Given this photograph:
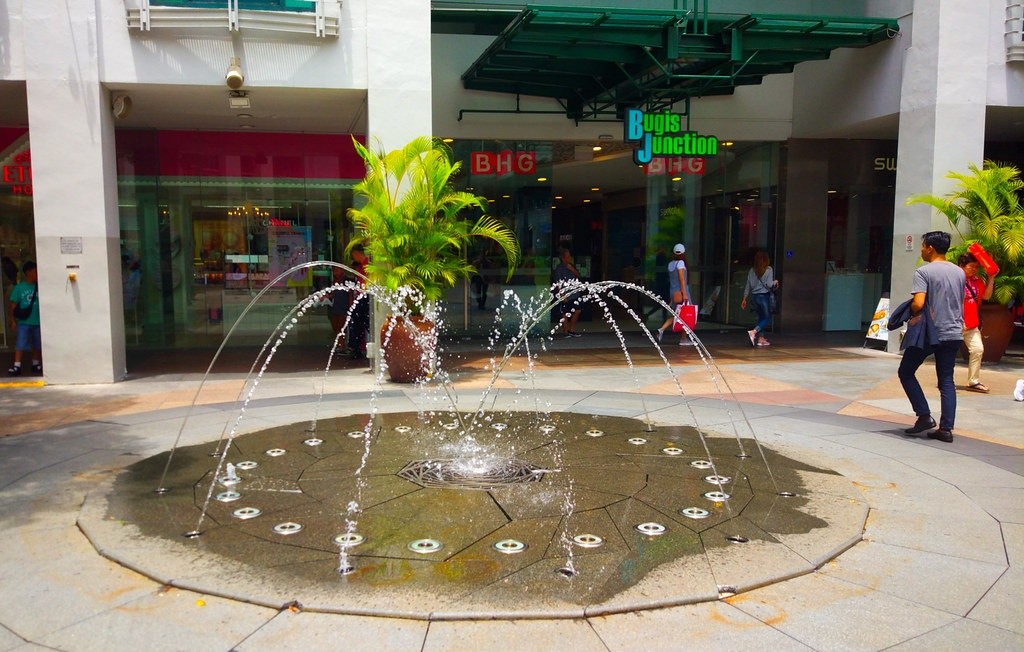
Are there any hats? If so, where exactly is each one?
[672,244,686,255]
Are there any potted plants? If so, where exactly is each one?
[904,154,1024,361]
[343,134,521,379]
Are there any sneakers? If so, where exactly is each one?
[655,327,664,342]
[746,330,756,346]
[757,340,771,347]
[678,338,694,346]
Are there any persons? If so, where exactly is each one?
[553,248,582,339]
[6,260,42,375]
[1014,379,1024,401]
[657,245,699,346]
[952,251,1000,394]
[883,231,965,443]
[218,251,227,267]
[120,242,142,326]
[740,250,779,347]
[1,256,18,348]
[325,263,353,353]
[228,251,243,289]
[642,251,670,323]
[314,265,323,292]
[352,261,361,278]
[345,243,369,360]
[199,246,222,324]
[471,250,491,310]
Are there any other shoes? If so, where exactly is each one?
[30,363,43,374]
[8,364,21,376]
[338,346,355,358]
[563,332,572,338]
[569,330,581,337]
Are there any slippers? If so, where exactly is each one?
[967,382,988,395]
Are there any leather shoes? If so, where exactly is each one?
[905,415,936,434]
[926,427,954,444]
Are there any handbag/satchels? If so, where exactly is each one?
[673,290,689,304]
[768,289,780,317]
[673,299,698,334]
[13,301,33,321]
